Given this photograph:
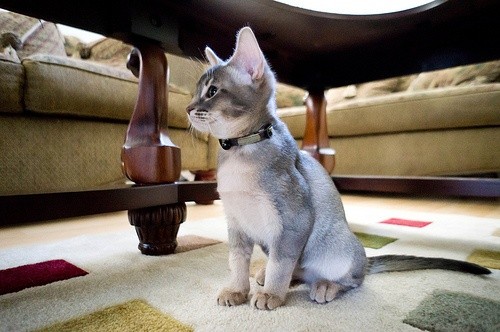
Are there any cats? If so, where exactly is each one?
[185,26,493,312]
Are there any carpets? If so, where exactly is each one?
[0,203,500,332]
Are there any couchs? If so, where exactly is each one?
[0,9,500,205]
